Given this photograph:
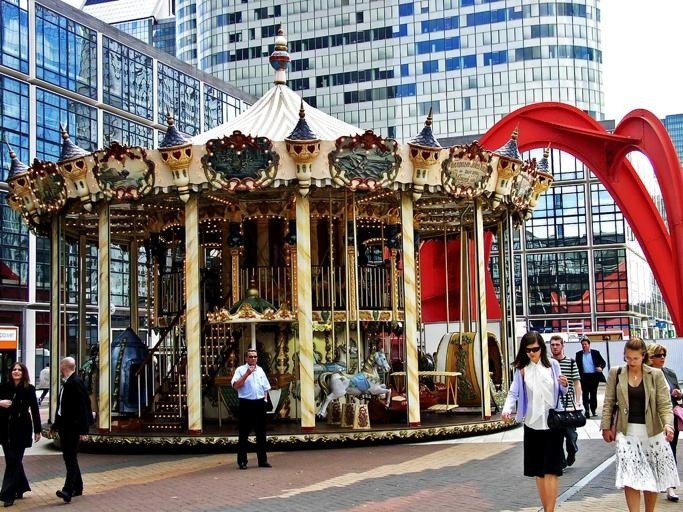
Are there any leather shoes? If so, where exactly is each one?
[4,493,23,507]
[667,490,679,502]
[585,412,590,419]
[239,463,247,470]
[591,409,598,416]
[56,491,71,502]
[259,464,272,467]
[72,490,82,497]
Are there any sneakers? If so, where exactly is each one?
[567,452,575,466]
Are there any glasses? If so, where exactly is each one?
[625,352,646,362]
[525,347,540,353]
[652,354,666,358]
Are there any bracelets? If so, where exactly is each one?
[264,399,268,403]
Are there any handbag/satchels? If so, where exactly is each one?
[610,404,619,441]
[673,404,683,431]
[547,409,586,429]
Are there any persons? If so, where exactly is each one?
[600,337,675,512]
[231,348,272,470]
[549,336,582,466]
[501,331,569,512]
[646,342,681,502]
[38,363,50,405]
[49,356,93,504]
[575,338,606,419]
[0,362,43,508]
